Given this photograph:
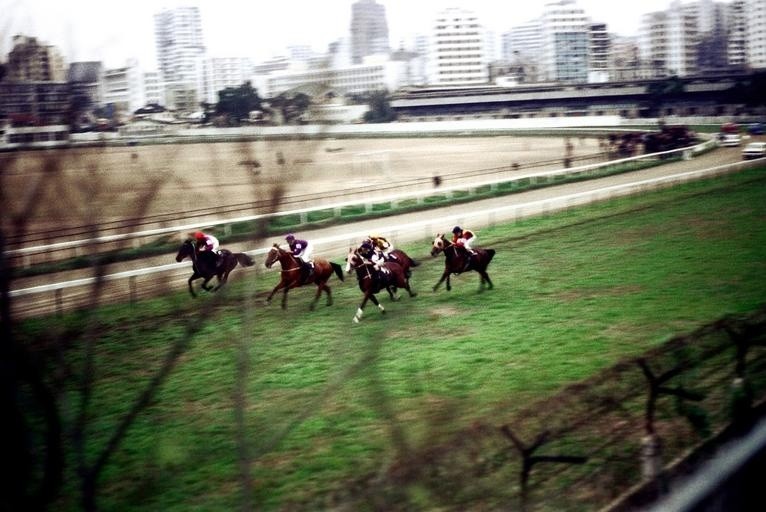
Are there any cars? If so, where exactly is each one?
[717,134,742,147]
[746,122,764,135]
[720,122,742,133]
[741,142,766,158]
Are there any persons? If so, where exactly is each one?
[285,235,315,269]
[362,240,385,271]
[193,231,224,269]
[368,236,393,261]
[446,226,476,254]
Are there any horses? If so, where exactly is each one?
[176,239,255,299]
[262,241,344,311]
[430,233,496,294]
[345,239,421,324]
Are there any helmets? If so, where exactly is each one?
[285,235,295,240]
[194,232,204,238]
[360,232,378,250]
[452,226,461,233]
